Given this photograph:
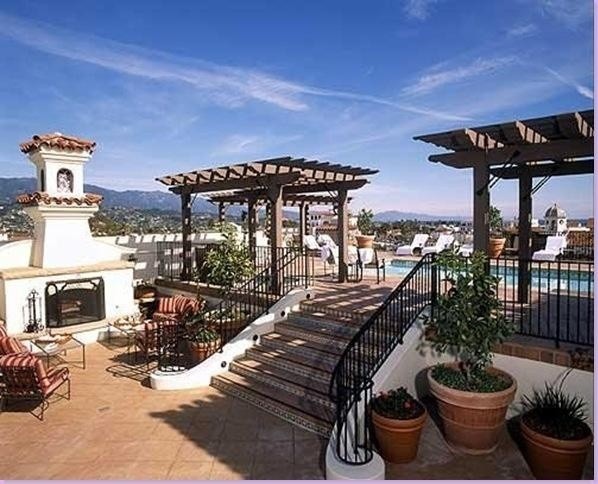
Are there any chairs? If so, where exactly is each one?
[0,324,71,421]
[505,236,532,258]
[532,236,567,261]
[304,234,385,284]
[395,234,474,257]
[133,296,206,371]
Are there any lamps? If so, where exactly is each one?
[27,288,44,333]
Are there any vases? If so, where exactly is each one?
[369,402,427,463]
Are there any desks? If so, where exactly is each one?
[30,333,86,369]
[108,316,146,362]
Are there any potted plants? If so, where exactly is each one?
[199,218,256,346]
[186,330,222,363]
[354,209,377,248]
[417,239,520,457]
[507,367,593,480]
[487,205,507,258]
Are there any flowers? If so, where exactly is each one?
[371,386,418,417]
